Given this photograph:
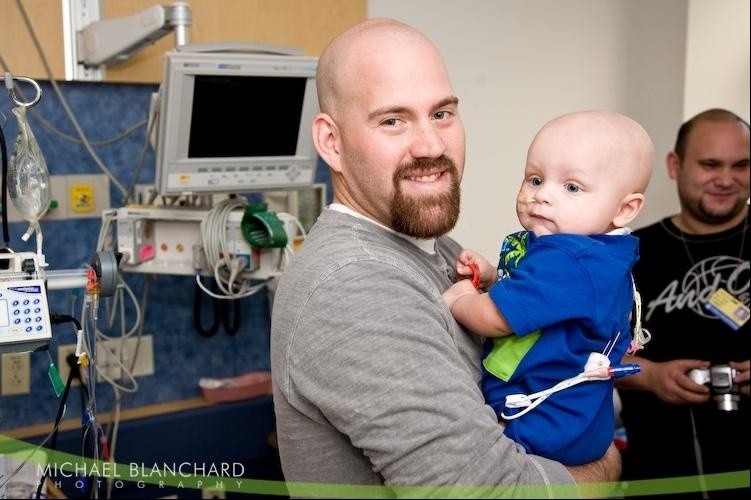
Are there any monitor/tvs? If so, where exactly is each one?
[154,50,322,196]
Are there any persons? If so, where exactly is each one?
[440,110,656,467]
[271,18,623,500]
[619,108,750,500]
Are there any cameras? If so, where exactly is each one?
[686,363,741,417]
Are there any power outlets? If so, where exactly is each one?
[97,340,122,383]
[58,343,89,387]
[1,351,30,396]
